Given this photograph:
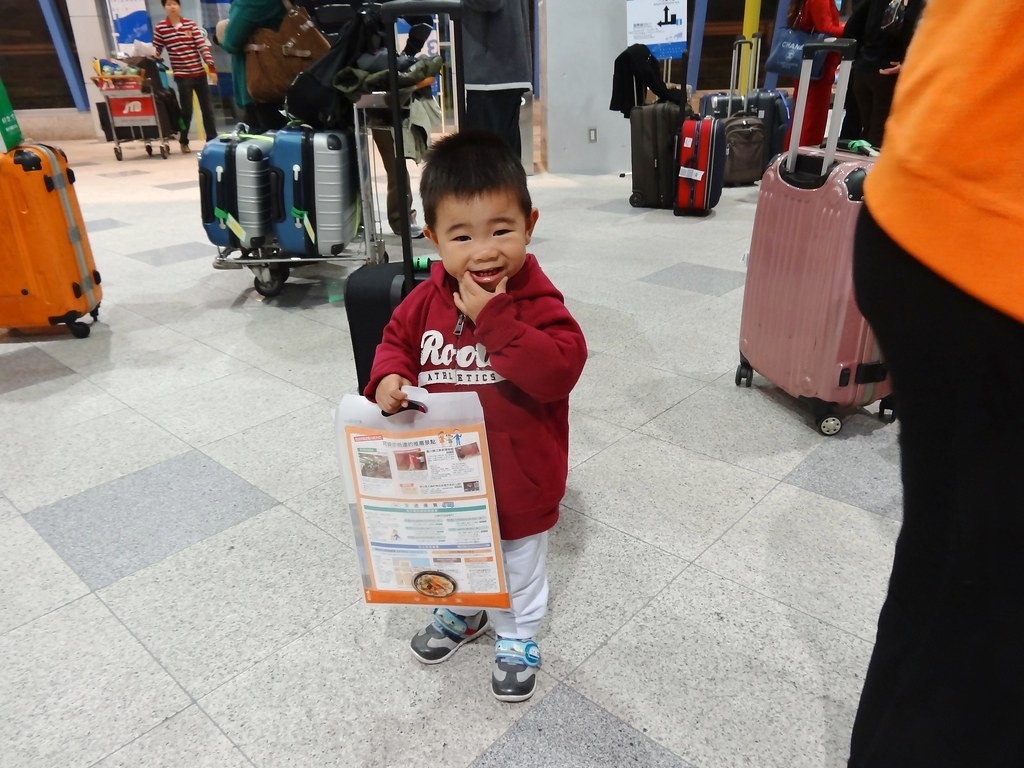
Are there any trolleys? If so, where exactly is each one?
[90,75,180,161]
[210,74,437,296]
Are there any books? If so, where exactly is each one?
[346,425,509,613]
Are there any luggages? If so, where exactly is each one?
[0,140,101,338]
[201,120,360,258]
[737,42,901,438]
[629,36,791,217]
[119,91,181,138]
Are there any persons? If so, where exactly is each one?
[213,0,426,272]
[457,1,534,162]
[360,124,587,705]
[144,0,217,154]
[782,0,848,153]
[849,0,1024,768]
[841,0,926,141]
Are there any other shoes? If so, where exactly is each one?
[397,225,427,238]
[181,141,190,153]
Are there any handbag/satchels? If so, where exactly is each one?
[242,0,334,105]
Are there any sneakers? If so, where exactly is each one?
[410,606,490,665]
[491,635,540,703]
